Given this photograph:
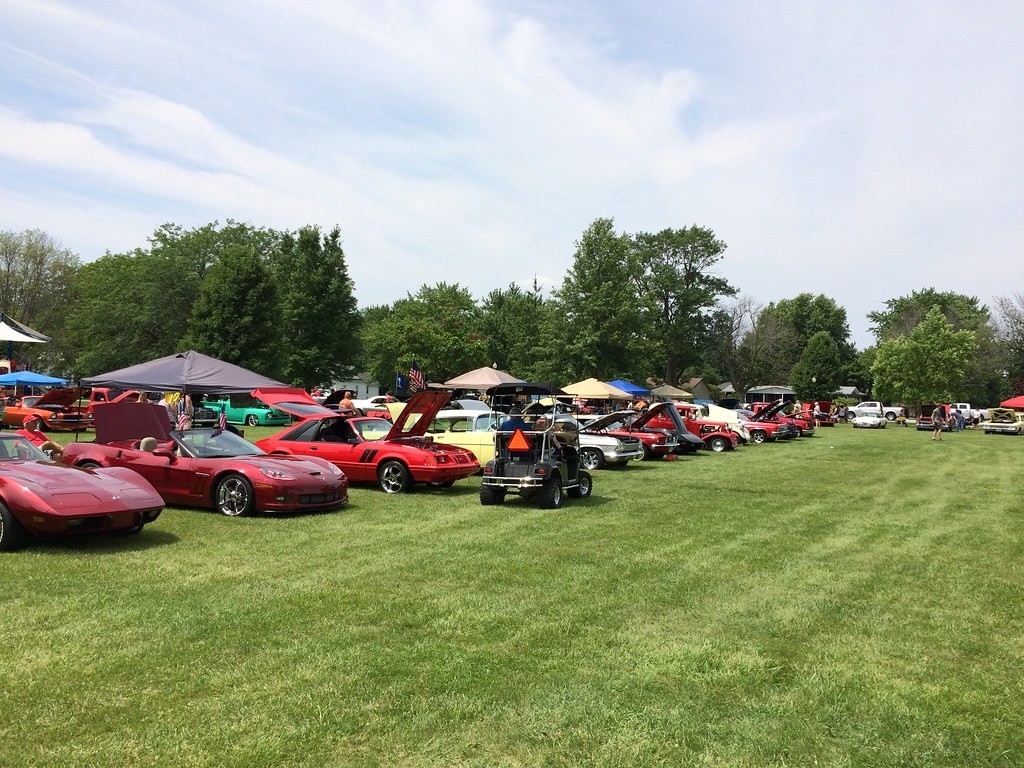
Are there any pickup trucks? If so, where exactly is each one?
[949,403,991,423]
[845,402,903,421]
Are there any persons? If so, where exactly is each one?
[338,391,362,417]
[972,408,980,431]
[13,415,64,463]
[386,391,397,403]
[613,400,634,412]
[500,407,532,431]
[536,419,564,460]
[310,387,322,397]
[134,390,194,430]
[778,398,848,428]
[930,403,946,440]
[948,412,965,433]
[897,405,908,428]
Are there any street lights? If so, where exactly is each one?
[812,377,816,401]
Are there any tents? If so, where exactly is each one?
[443,366,528,409]
[76,349,292,443]
[549,377,633,441]
[650,385,694,418]
[605,380,651,412]
[0,371,69,396]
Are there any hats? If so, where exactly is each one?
[23,415,39,424]
[538,416,546,423]
[814,402,819,405]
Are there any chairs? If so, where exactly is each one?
[140,436,156,452]
[321,427,336,442]
[176,438,194,458]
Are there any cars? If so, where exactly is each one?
[540,410,643,470]
[916,404,952,431]
[648,403,752,452]
[316,390,400,416]
[851,411,887,428]
[88,387,219,428]
[362,398,562,468]
[805,402,835,427]
[982,408,1024,436]
[745,402,815,438]
[2,388,94,432]
[584,402,705,461]
[200,394,291,427]
[730,399,791,445]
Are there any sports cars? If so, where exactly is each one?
[61,403,348,517]
[0,432,166,552]
[250,387,481,494]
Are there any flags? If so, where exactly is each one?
[397,374,407,390]
[408,359,428,392]
